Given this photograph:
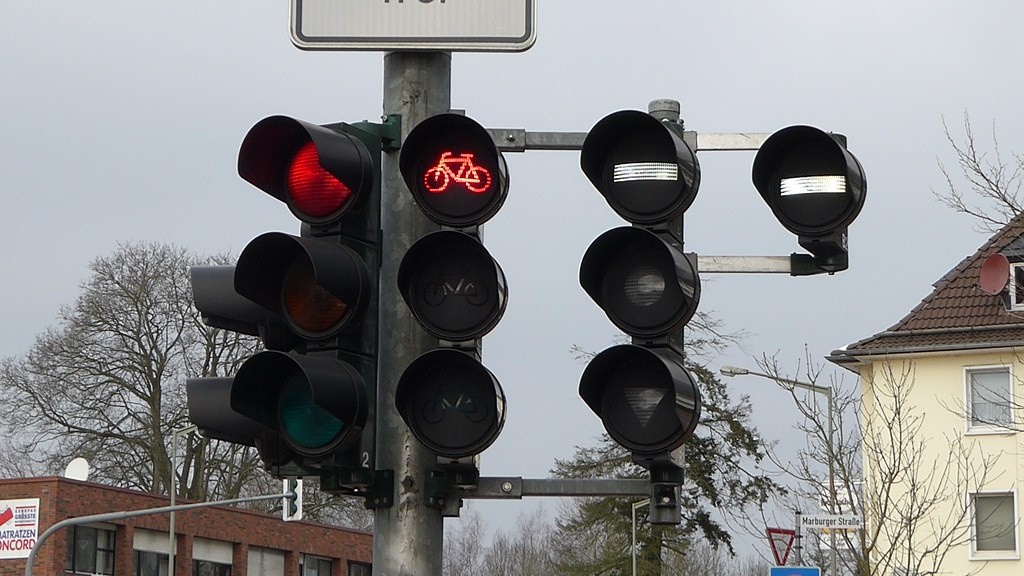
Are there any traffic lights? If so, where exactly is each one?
[185,265,294,469]
[399,112,509,457]
[228,115,376,464]
[751,125,869,239]
[581,109,702,455]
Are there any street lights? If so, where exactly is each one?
[720,364,837,575]
